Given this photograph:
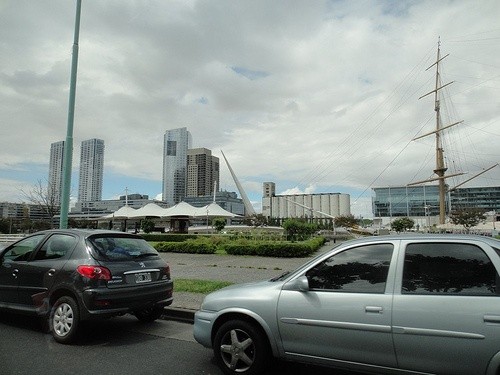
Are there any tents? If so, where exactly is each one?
[102,202,244,233]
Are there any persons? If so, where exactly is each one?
[334,231,337,243]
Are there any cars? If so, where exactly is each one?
[193,234,500,375]
[0,228,174,344]
